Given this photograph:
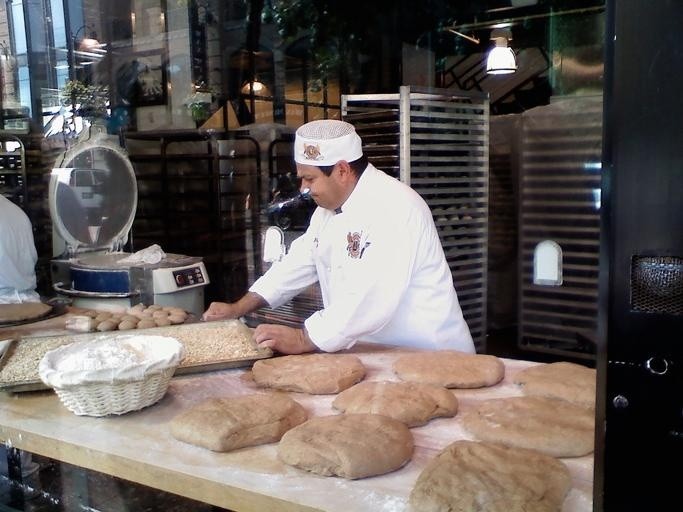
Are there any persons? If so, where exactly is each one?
[196,119,477,359]
[0,194,42,306]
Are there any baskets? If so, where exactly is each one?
[37,334,182,418]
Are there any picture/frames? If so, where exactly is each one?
[109,47,169,109]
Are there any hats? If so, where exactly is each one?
[293,118,363,168]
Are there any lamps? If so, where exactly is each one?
[486,25,519,76]
